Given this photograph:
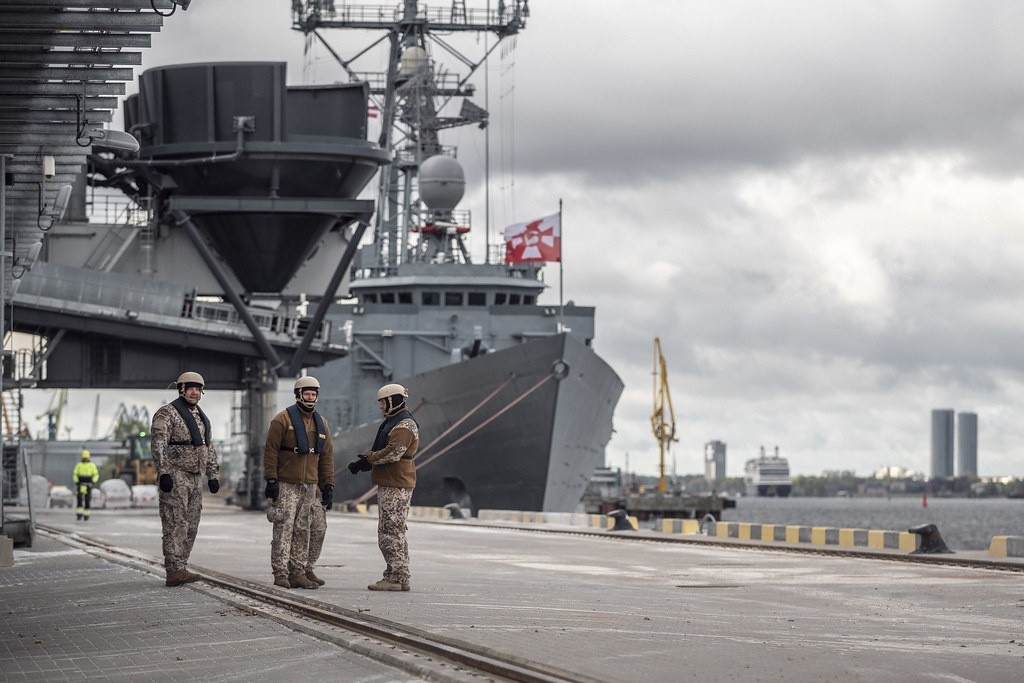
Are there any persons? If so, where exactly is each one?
[73,450,99,520]
[150,372,220,587]
[264,377,336,589]
[348,383,419,592]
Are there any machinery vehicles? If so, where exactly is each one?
[113,434,158,486]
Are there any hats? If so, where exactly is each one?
[81,450,90,459]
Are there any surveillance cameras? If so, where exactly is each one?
[42,155,56,179]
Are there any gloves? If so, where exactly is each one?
[208,480,219,493]
[348,455,372,474]
[159,474,173,493]
[320,484,334,510]
[265,478,279,502]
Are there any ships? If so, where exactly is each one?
[745,446,792,497]
[291,0,627,510]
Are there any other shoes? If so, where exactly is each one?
[367,578,402,591]
[274,575,291,588]
[77,515,82,520]
[305,571,325,586]
[83,516,88,520]
[401,580,410,591]
[166,566,201,586]
[288,573,319,589]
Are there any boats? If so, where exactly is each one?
[578,338,735,522]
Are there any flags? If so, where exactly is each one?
[504,212,561,263]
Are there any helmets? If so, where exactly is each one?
[294,376,320,390]
[177,372,204,387]
[377,384,408,400]
